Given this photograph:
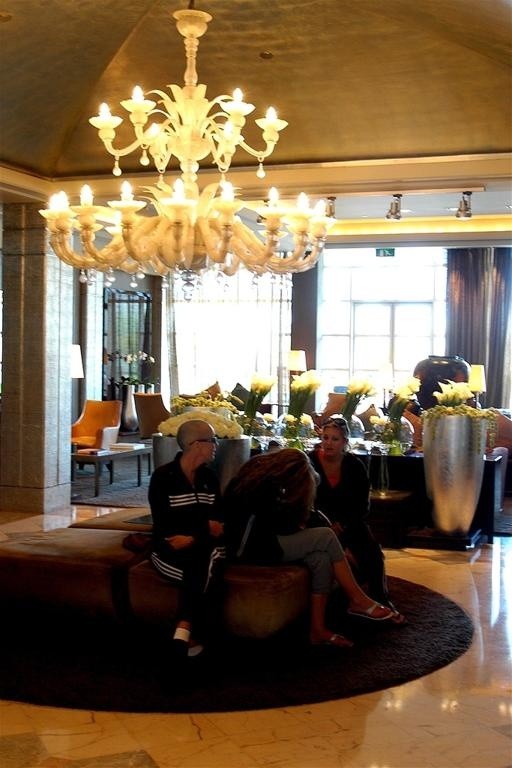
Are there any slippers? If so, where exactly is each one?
[311,634,354,646]
[347,602,408,624]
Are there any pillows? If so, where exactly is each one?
[204,381,221,401]
[226,382,251,403]
[401,410,421,446]
[467,365,486,410]
[322,393,348,415]
[358,404,380,430]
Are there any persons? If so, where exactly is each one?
[306,419,406,626]
[148,419,228,670]
[220,447,394,648]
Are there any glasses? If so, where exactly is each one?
[189,437,219,446]
[321,417,349,432]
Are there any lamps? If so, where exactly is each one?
[255,200,269,224]
[285,350,307,376]
[385,194,404,219]
[38,1,337,302]
[454,191,474,217]
[72,345,85,379]
[325,197,336,220]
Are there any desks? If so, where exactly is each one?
[73,441,154,497]
[250,435,503,545]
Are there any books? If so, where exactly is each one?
[77,448,110,454]
[109,442,146,450]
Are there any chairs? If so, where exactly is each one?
[133,393,174,459]
[485,406,512,459]
[73,400,124,484]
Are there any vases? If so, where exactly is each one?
[123,383,155,431]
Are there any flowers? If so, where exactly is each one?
[111,347,160,389]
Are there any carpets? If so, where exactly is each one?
[1,572,475,714]
